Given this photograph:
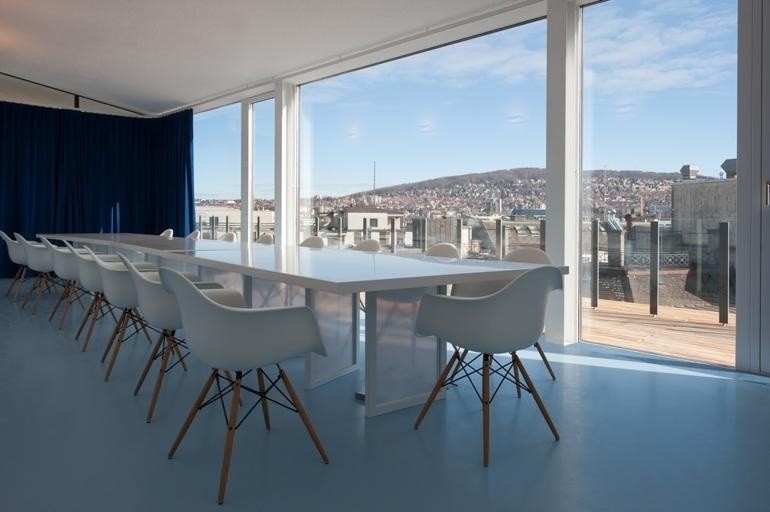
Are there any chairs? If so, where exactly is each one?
[40,235,117,327]
[1,232,62,301]
[82,244,190,382]
[14,232,85,315]
[413,266,564,468]
[158,228,461,310]
[158,267,330,504]
[114,250,245,422]
[62,240,152,352]
[451,245,555,401]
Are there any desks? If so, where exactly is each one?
[37,232,564,419]
